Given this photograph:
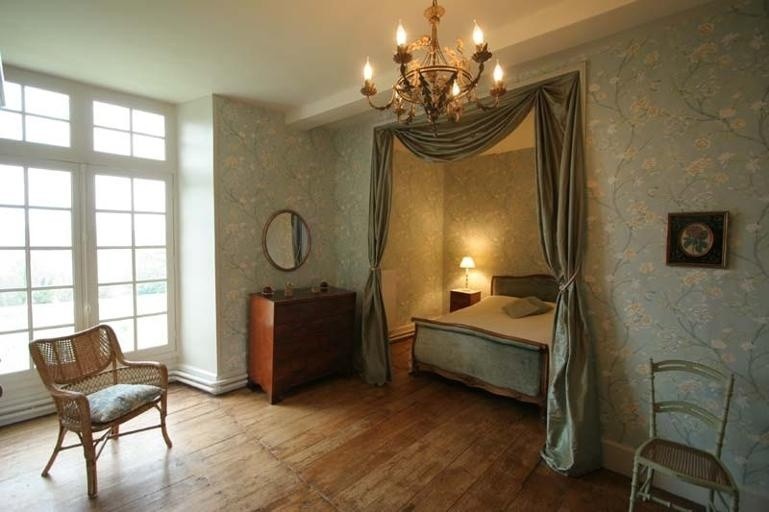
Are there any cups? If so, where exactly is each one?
[262,281,331,296]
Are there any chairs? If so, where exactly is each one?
[27,322,173,500]
[627,356,740,512]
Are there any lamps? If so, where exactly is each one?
[358,2,508,140]
[458,255,476,292]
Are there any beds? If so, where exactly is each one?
[408,274,560,426]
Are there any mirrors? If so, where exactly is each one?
[261,208,313,273]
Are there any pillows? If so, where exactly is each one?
[501,294,555,320]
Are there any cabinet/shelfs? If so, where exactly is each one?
[246,285,358,406]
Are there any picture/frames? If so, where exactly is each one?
[664,209,731,270]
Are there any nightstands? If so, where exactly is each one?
[449,288,482,313]
